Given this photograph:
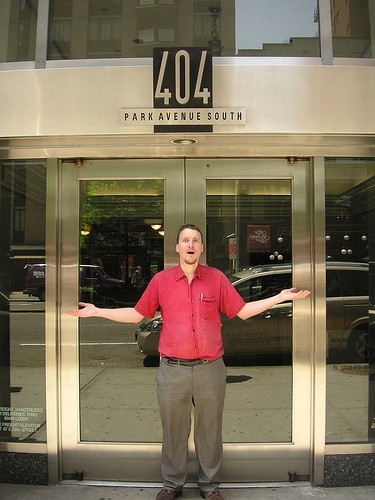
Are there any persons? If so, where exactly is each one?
[66,224,313,500]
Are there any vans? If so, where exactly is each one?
[133,258,371,360]
[22,263,124,302]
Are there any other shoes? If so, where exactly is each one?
[199,489,224,500]
[155,487,183,500]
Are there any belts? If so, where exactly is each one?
[163,355,207,367]
